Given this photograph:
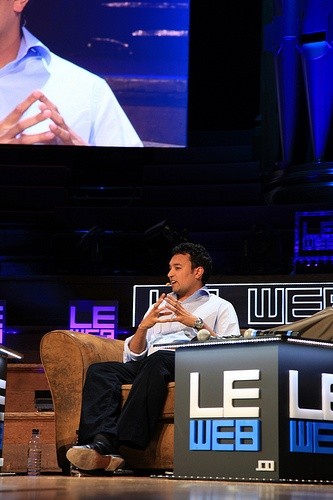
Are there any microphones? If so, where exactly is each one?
[244,329,300,338]
[197,329,226,341]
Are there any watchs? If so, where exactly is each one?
[193,317,204,332]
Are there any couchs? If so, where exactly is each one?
[39,329,175,475]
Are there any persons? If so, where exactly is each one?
[65,243,240,476]
[0,0,144,147]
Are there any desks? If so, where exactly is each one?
[175,336,333,483]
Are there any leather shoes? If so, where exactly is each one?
[67,440,125,472]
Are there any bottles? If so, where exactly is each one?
[71,430,82,477]
[28,429,42,475]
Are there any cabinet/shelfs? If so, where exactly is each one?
[1,364,62,473]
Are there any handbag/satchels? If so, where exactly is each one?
[267,305,333,341]
